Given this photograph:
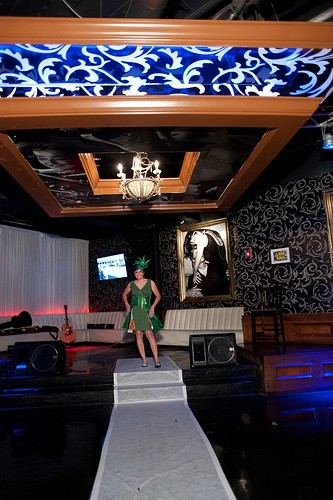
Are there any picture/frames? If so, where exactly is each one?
[177,217,235,302]
[269,246,292,265]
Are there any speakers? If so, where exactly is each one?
[189,332,239,369]
[11,341,66,378]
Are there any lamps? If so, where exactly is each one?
[116,152,162,202]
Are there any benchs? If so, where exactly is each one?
[0,307,246,352]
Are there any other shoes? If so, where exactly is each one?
[142,363,148,367]
[155,361,161,368]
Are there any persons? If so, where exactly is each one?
[184,230,230,298]
[122,254,165,368]
[7,127,240,208]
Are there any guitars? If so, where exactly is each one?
[60,305,76,343]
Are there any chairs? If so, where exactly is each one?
[251,286,287,351]
